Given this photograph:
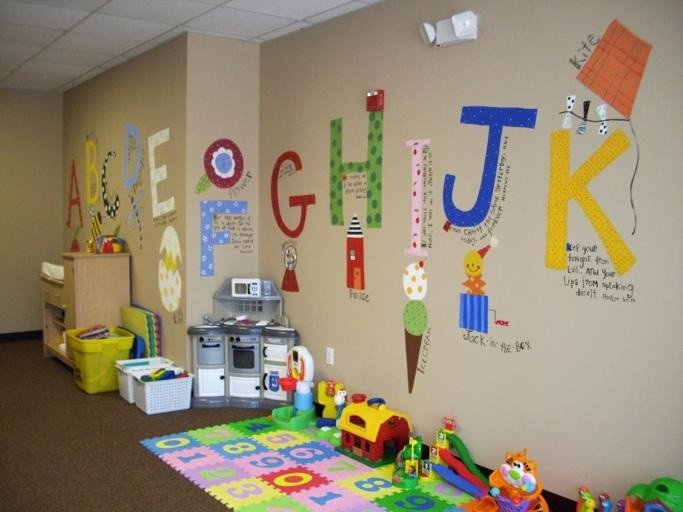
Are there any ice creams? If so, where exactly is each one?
[402,261,428,394]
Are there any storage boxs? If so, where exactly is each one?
[114,358,174,404]
[65,322,135,396]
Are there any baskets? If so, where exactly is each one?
[115,357,193,416]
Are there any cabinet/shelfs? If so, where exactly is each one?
[41,251,131,369]
[188,324,301,411]
